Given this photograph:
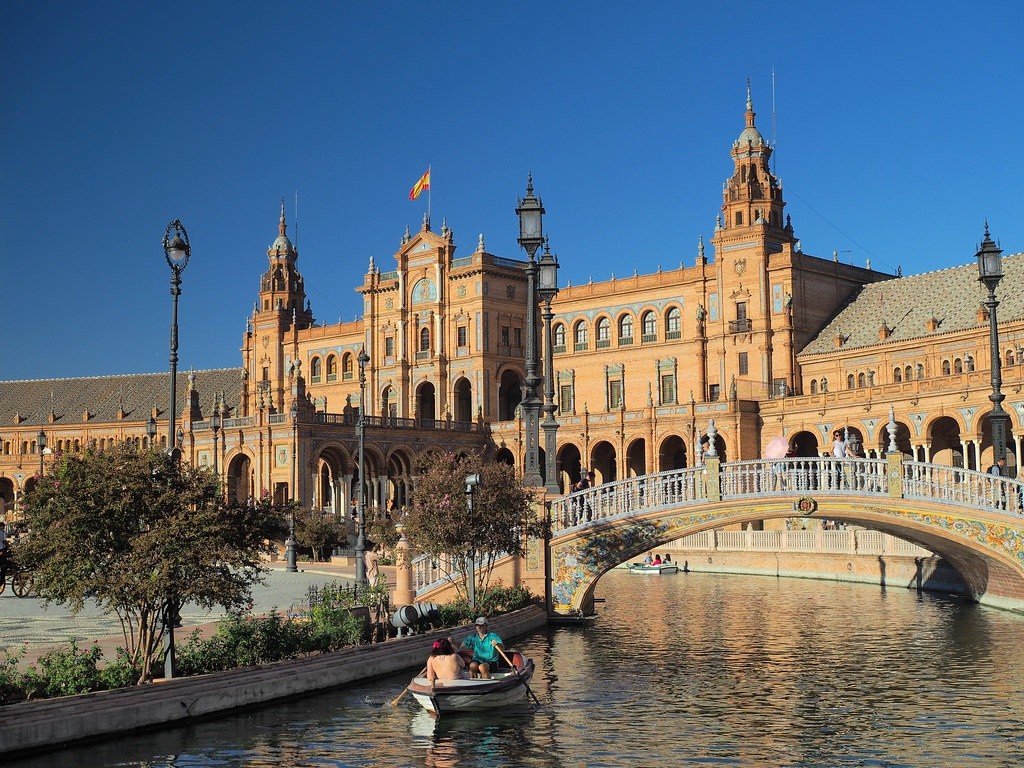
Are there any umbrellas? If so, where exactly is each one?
[766,437,789,458]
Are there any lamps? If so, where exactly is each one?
[778,418,783,422]
[910,402,917,406]
[1012,389,1020,393]
[961,396,966,401]
[819,413,824,416]
[863,408,870,412]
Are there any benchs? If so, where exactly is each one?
[461,667,512,680]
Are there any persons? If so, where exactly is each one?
[573,472,595,522]
[0,522,10,550]
[427,638,465,679]
[830,430,860,489]
[644,552,652,564]
[652,554,661,566]
[663,554,671,563]
[991,457,1007,509]
[773,462,786,491]
[362,540,385,611]
[1018,467,1024,510]
[447,617,505,679]
[702,442,722,500]
[819,519,848,530]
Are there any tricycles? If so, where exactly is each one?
[0,540,48,599]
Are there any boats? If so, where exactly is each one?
[406,648,536,717]
[625,562,679,574]
[409,697,539,745]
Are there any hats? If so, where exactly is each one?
[474,617,489,627]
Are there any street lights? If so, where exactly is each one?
[161,215,193,462]
[536,236,564,500]
[37,427,47,484]
[972,216,1010,478]
[210,408,223,478]
[512,171,548,490]
[283,400,302,573]
[144,411,158,455]
[355,341,371,595]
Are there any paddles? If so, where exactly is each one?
[389,666,427,708]
[494,644,541,706]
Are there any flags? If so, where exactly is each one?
[409,170,429,200]
